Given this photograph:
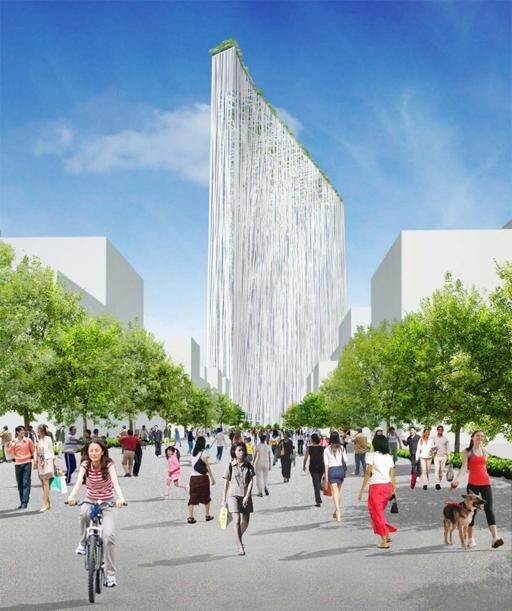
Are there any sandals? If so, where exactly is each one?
[206,516,214,521]
[187,517,197,523]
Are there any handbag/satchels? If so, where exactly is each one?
[4,442,15,461]
[323,479,332,496]
[274,439,285,458]
[219,506,234,530]
[410,464,418,489]
[447,463,454,481]
[320,474,327,491]
[390,497,399,513]
[341,455,347,471]
[194,450,207,475]
[50,473,68,493]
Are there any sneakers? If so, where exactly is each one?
[424,485,427,490]
[76,543,86,555]
[103,575,118,588]
[466,537,476,548]
[491,536,505,549]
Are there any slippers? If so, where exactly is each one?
[377,538,392,548]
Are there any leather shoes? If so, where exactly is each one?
[124,473,131,477]
[436,484,442,490]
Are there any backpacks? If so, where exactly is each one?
[272,437,277,446]
